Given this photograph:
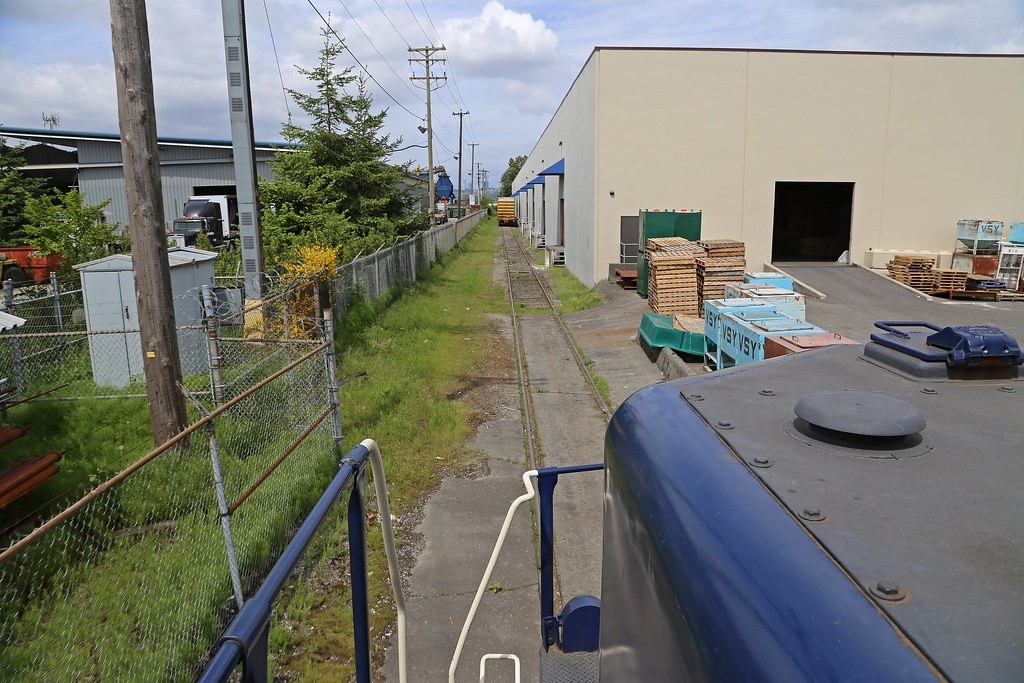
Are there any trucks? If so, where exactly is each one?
[164,194,239,255]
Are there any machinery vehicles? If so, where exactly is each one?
[0,246,66,289]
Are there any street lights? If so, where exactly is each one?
[454,153,461,220]
[417,126,436,230]
[468,173,473,213]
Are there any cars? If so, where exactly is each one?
[435,210,447,225]
